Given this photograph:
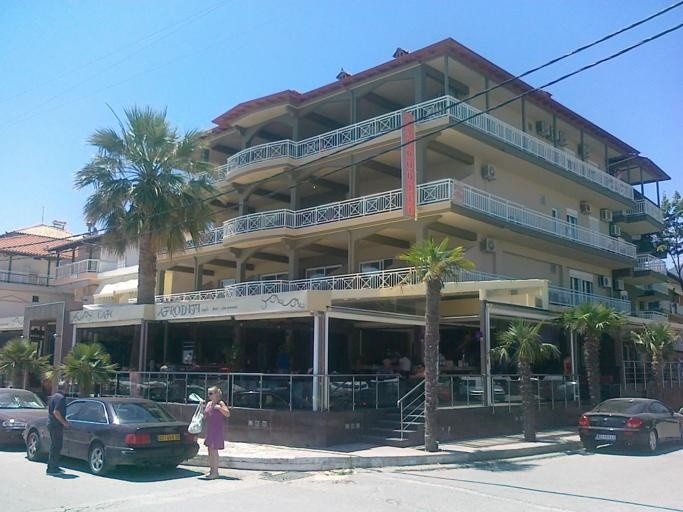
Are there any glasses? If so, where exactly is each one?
[209,392,213,395]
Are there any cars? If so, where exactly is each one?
[577,397,682,454]
[22,395,200,476]
[2,387,49,446]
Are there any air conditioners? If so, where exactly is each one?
[599,275,613,288]
[610,224,621,236]
[600,209,613,223]
[483,163,496,181]
[615,279,625,290]
[581,202,591,214]
[481,237,497,254]
[537,120,568,146]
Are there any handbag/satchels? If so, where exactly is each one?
[188,413,204,434]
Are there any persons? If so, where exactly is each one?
[46,381,72,475]
[199,385,231,479]
[382,356,393,369]
[398,353,412,379]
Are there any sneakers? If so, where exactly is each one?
[206,473,219,479]
[46,467,64,476]
